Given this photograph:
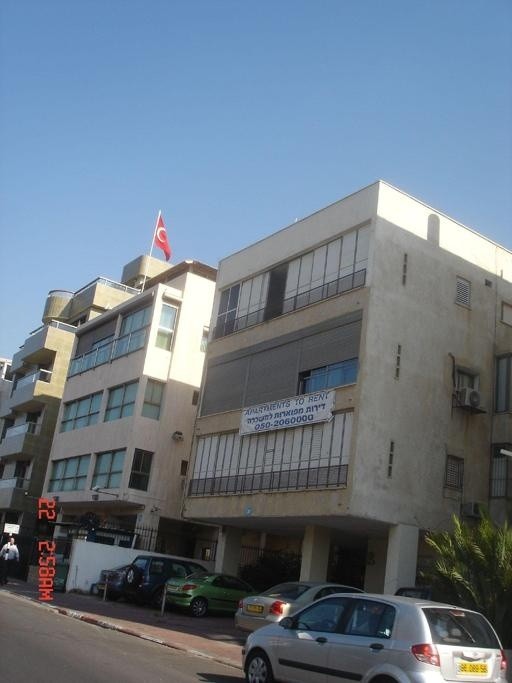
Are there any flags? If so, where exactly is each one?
[154,217,174,261]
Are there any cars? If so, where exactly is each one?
[161,571,261,618]
[233,577,370,635]
[240,590,509,683]
[96,561,130,599]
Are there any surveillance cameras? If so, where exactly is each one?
[92,485,100,492]
[24,491,31,496]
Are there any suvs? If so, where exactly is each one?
[398,582,496,619]
[121,553,207,608]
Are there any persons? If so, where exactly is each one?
[0,537,20,582]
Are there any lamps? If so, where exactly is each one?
[91,485,120,497]
[52,495,60,501]
[23,488,41,500]
[92,494,99,500]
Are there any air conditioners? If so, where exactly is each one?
[460,499,489,520]
[454,385,490,414]
